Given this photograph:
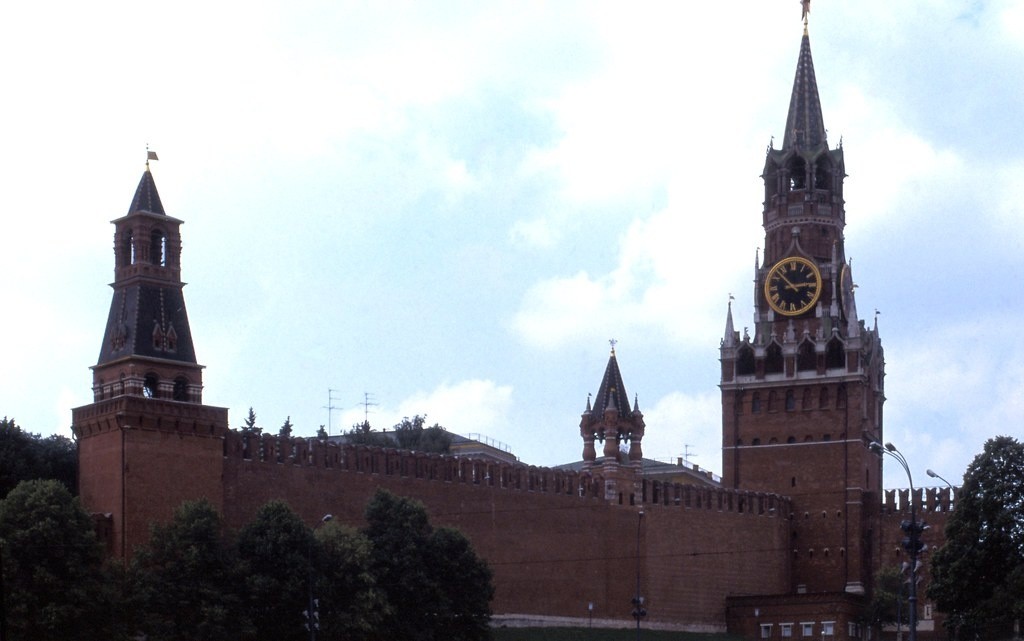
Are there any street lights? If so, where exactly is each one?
[631,510,647,630]
[867,442,931,641]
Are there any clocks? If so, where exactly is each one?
[840,264,854,320]
[764,257,823,316]
